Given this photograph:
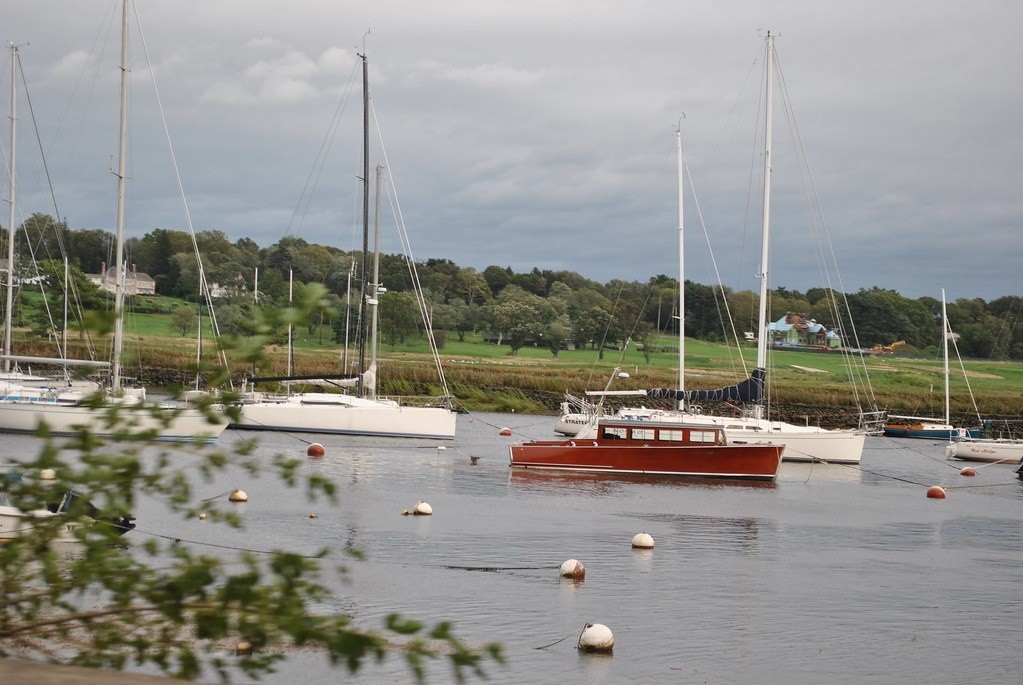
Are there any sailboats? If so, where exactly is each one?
[507,23,1022,486]
[1,0,459,447]
